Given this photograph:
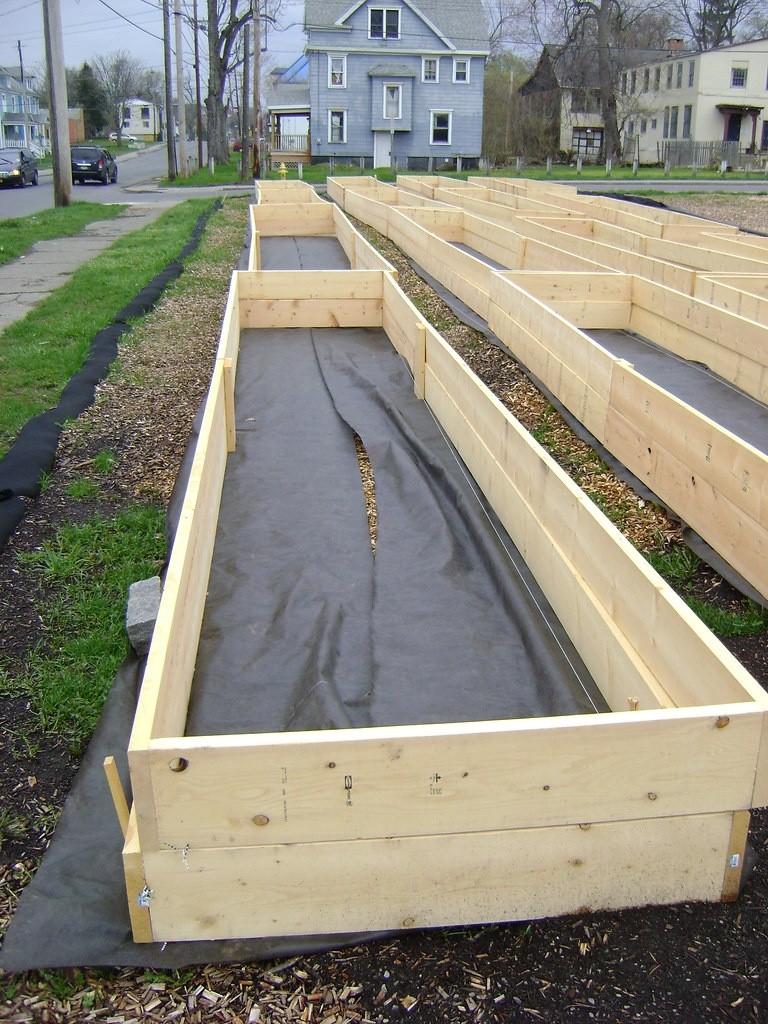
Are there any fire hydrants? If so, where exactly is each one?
[277,162,289,181]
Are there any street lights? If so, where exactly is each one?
[172,10,205,171]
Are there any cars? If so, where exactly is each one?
[109,131,138,144]
[0,146,40,188]
[232,139,256,154]
[70,144,118,186]
